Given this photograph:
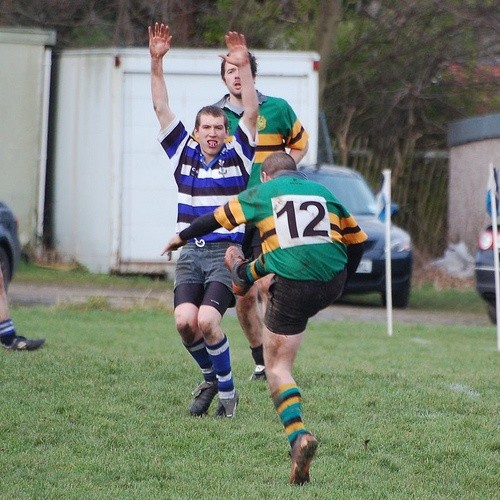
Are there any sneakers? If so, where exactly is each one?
[225,246,251,297]
[1,336,46,351]
[289,433,318,484]
[186,378,239,419]
[251,364,267,384]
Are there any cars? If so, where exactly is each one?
[301,164,413,307]
[474,223,500,325]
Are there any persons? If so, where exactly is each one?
[192,52,309,384]
[0,201,45,350]
[148,22,258,418]
[161,152,369,484]
